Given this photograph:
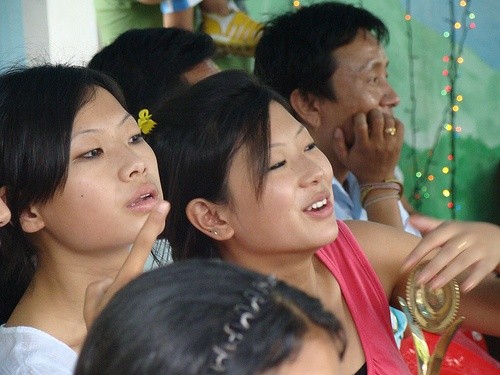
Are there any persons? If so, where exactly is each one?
[0,63,178,375]
[89,25,225,112]
[74,259,346,375]
[253,0,424,237]
[148,69,500,375]
[137,1,266,56]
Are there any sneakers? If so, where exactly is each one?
[199,1,264,59]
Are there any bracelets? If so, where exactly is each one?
[359,179,403,207]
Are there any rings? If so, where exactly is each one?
[385,127,397,135]
